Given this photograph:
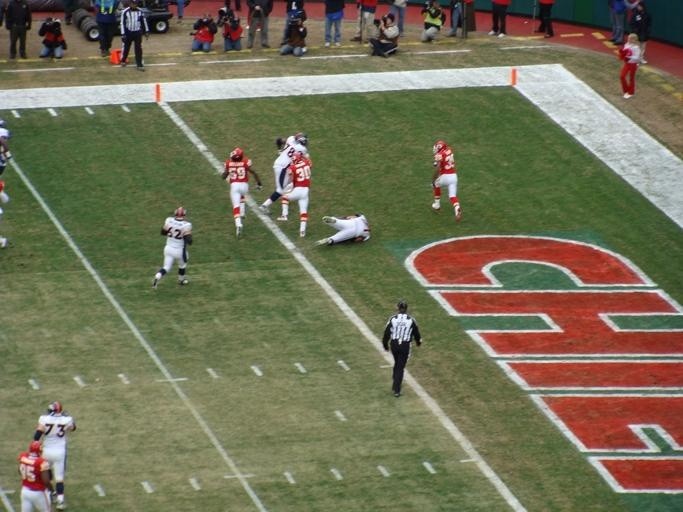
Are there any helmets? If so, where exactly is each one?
[229,148,243,161]
[431,140,447,156]
[397,301,407,309]
[29,440,42,457]
[294,132,308,146]
[291,150,303,165]
[174,206,186,217]
[47,400,62,414]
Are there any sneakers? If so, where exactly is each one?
[432,203,442,211]
[390,387,400,397]
[321,215,337,224]
[47,491,65,510]
[234,204,306,238]
[176,279,188,286]
[312,238,330,249]
[453,205,462,221]
[488,30,506,39]
[622,92,633,100]
[152,278,157,290]
[638,59,648,66]
[301,42,342,54]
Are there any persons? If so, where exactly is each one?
[34,400,76,509]
[17,440,53,512]
[219,148,263,240]
[0,0,552,69]
[149,206,194,290]
[313,212,372,246]
[381,298,423,397]
[0,118,10,249]
[431,140,461,222]
[613,32,641,100]
[258,132,313,237]
